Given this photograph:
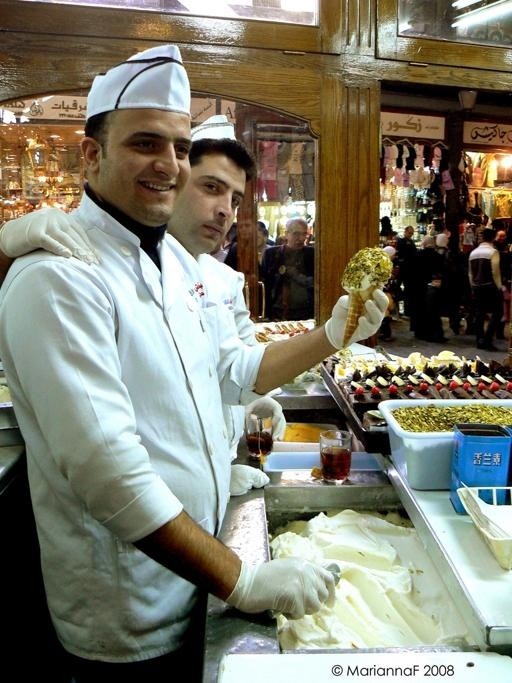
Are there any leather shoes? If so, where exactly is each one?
[483,340,498,352]
[477,338,484,349]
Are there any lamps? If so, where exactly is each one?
[451,0,512,35]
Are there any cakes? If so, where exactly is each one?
[322,350,512,431]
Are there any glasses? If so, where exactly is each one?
[408,231,414,235]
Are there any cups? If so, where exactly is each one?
[245,410,273,458]
[319,430,352,484]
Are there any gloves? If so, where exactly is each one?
[244,395,287,441]
[225,559,335,618]
[0,207,104,265]
[325,289,388,349]
[228,463,271,497]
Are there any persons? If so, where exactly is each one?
[396,226,417,317]
[445,237,473,336]
[0,115,285,465]
[258,219,315,320]
[413,235,445,343]
[468,229,510,351]
[224,220,274,271]
[253,228,273,271]
[494,229,511,342]
[1,46,389,683]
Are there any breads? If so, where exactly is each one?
[340,247,393,350]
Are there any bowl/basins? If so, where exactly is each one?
[274,422,342,451]
[378,398,512,490]
[261,451,383,474]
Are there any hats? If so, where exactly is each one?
[192,114,235,141]
[86,45,191,119]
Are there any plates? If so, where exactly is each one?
[319,359,512,454]
